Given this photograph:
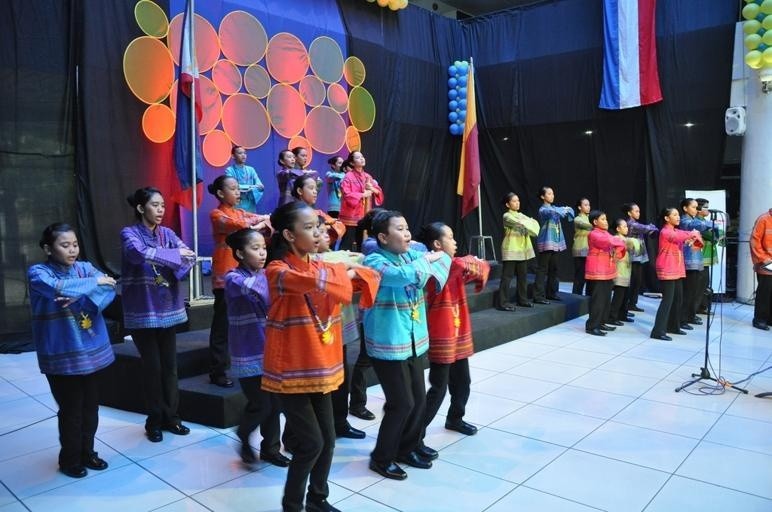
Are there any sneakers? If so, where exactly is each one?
[348,407,376,420]
[304,498,342,512]
[238,443,257,464]
[496,295,561,312]
[753,321,769,330]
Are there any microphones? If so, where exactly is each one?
[702,207,722,215]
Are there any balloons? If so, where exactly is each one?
[447,61,477,134]
[741,0,772,70]
[366,1,409,12]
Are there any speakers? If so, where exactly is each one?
[724,107,746,135]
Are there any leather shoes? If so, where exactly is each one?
[210,373,234,388]
[369,458,408,481]
[336,424,367,439]
[650,315,704,342]
[144,421,190,443]
[444,419,478,436]
[59,451,108,478]
[399,444,440,470]
[259,450,291,467]
[585,305,645,337]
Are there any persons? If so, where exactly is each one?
[413,221,491,460]
[259,201,384,511]
[535,185,575,305]
[585,197,729,342]
[494,191,541,312]
[207,144,431,467]
[119,186,199,443]
[26,223,119,480]
[363,209,454,480]
[570,196,594,295]
[748,208,771,330]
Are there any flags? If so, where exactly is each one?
[456,65,481,220]
[596,1,664,111]
[172,0,205,191]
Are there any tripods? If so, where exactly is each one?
[675,220,748,394]
